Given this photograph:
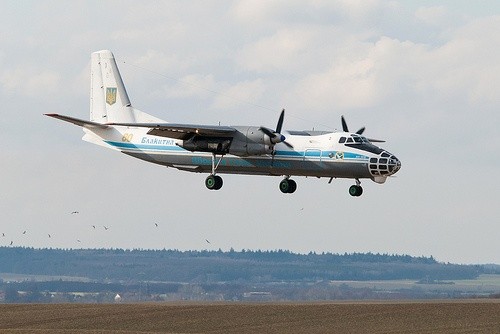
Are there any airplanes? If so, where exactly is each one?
[43,49,403,197]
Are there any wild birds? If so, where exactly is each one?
[2,210,210,245]
